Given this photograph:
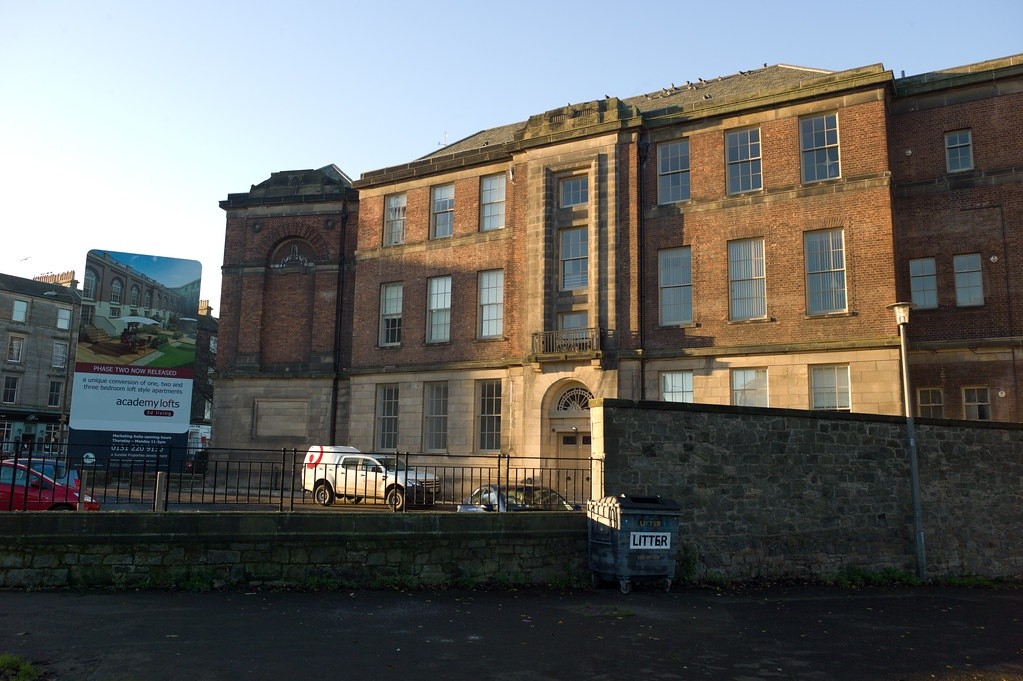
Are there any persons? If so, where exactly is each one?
[121,327,139,346]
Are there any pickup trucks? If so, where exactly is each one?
[300,444,440,511]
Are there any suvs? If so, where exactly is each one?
[0,459,82,491]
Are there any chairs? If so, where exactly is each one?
[120,335,152,355]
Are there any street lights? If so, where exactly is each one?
[885,301,929,584]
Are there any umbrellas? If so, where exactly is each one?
[115,315,160,338]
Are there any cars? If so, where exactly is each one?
[0,461,100,510]
[457,482,583,512]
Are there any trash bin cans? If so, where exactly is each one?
[585,494,684,594]
